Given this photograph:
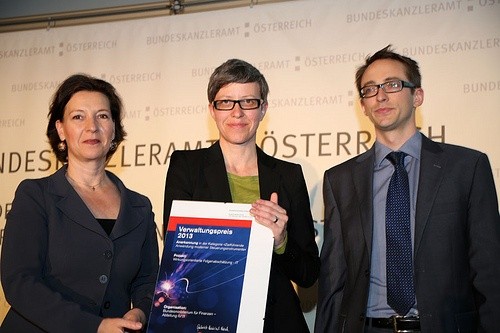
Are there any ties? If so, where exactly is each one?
[386,151,417,317]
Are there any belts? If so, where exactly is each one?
[366,316,421,333]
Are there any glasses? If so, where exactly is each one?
[212,98,263,112]
[359,79,419,99]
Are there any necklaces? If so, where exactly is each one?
[68,172,106,191]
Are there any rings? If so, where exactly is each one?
[273,216,279,223]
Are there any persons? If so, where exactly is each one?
[0,74,160,333]
[313,45,500,333]
[161,59,319,333]
